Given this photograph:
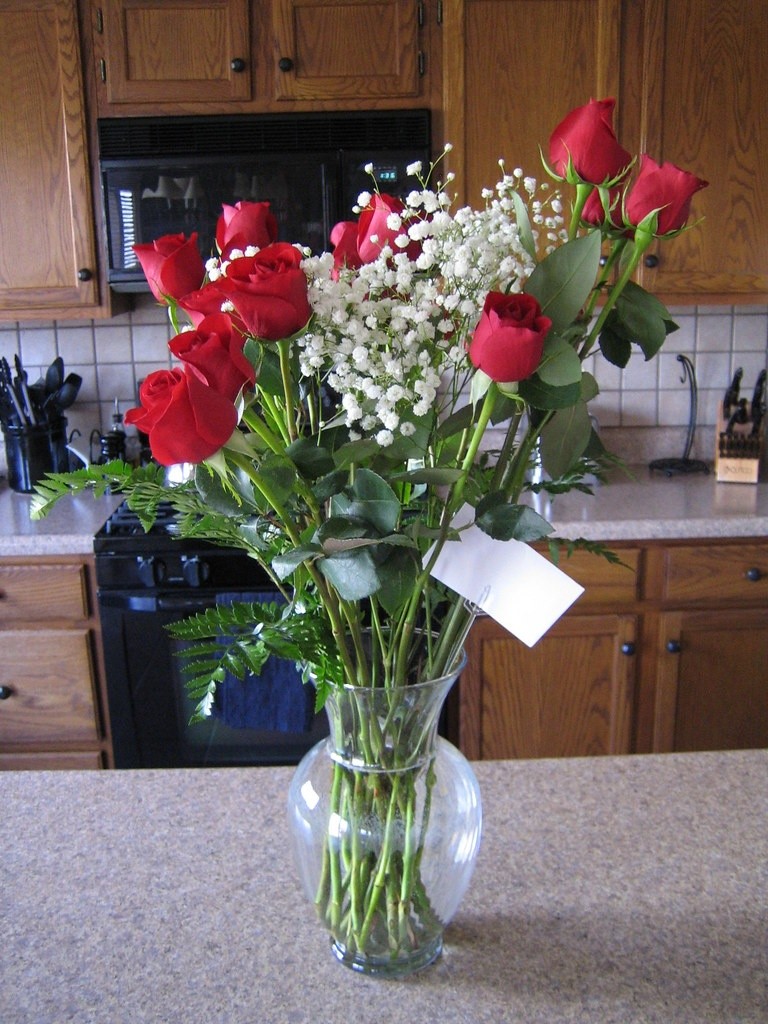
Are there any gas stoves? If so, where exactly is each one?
[90,382,300,594]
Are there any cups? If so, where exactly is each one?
[0,418,70,494]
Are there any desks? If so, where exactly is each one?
[0,747,767,1022]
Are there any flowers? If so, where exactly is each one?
[29,98,702,682]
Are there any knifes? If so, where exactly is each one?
[717,366,768,457]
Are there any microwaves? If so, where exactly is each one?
[95,110,437,295]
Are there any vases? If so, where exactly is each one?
[291,628,484,979]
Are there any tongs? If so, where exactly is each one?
[2,355,38,426]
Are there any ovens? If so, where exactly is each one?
[96,584,361,767]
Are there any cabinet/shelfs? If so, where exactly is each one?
[1,552,126,768]
[460,544,768,758]
[0,0,108,321]
[86,1,434,113]
[448,0,768,308]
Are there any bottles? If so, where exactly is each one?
[98,410,142,496]
